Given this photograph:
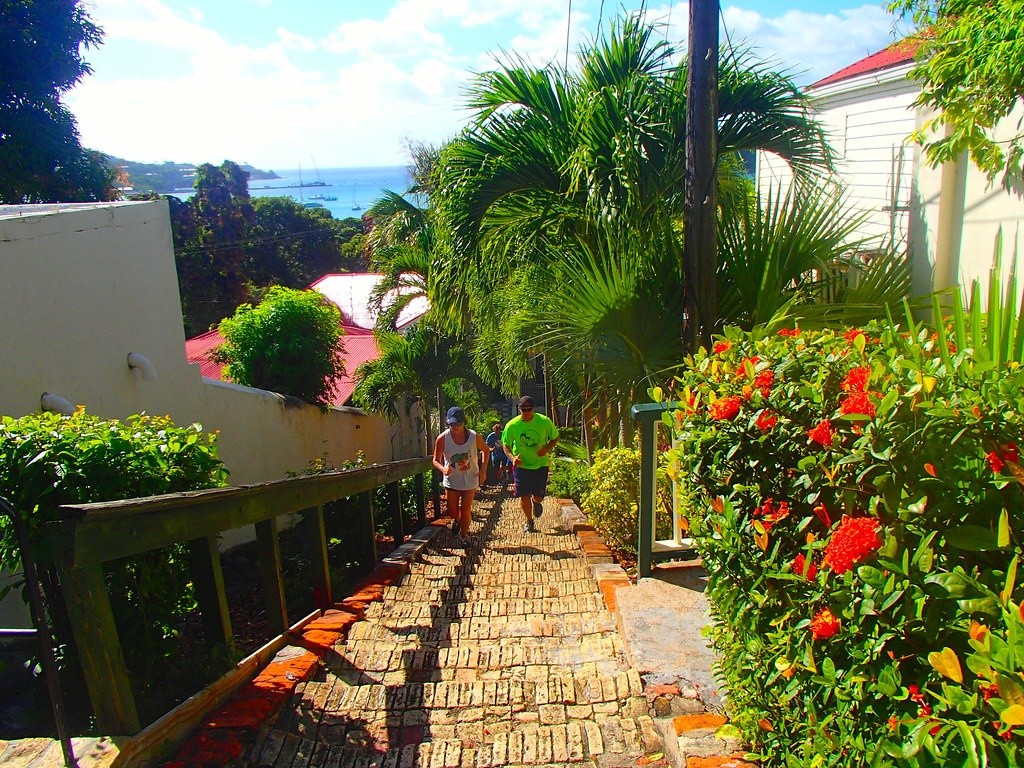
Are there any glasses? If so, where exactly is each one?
[521,407,533,412]
[449,422,463,426]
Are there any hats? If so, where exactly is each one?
[517,396,534,408]
[446,407,464,424]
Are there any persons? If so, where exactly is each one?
[432,407,490,547]
[486,424,514,495]
[501,396,560,532]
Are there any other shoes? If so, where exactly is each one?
[525,519,535,533]
[531,500,543,517]
[451,523,460,534]
